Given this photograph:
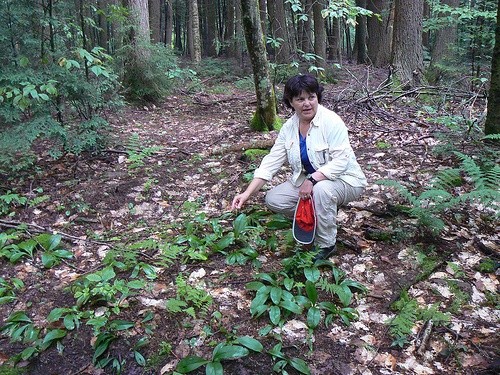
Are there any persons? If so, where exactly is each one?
[231,75,367,262]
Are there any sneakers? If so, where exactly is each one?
[310,245,338,263]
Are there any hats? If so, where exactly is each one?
[292,194,317,245]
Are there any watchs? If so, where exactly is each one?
[306,174,318,186]
